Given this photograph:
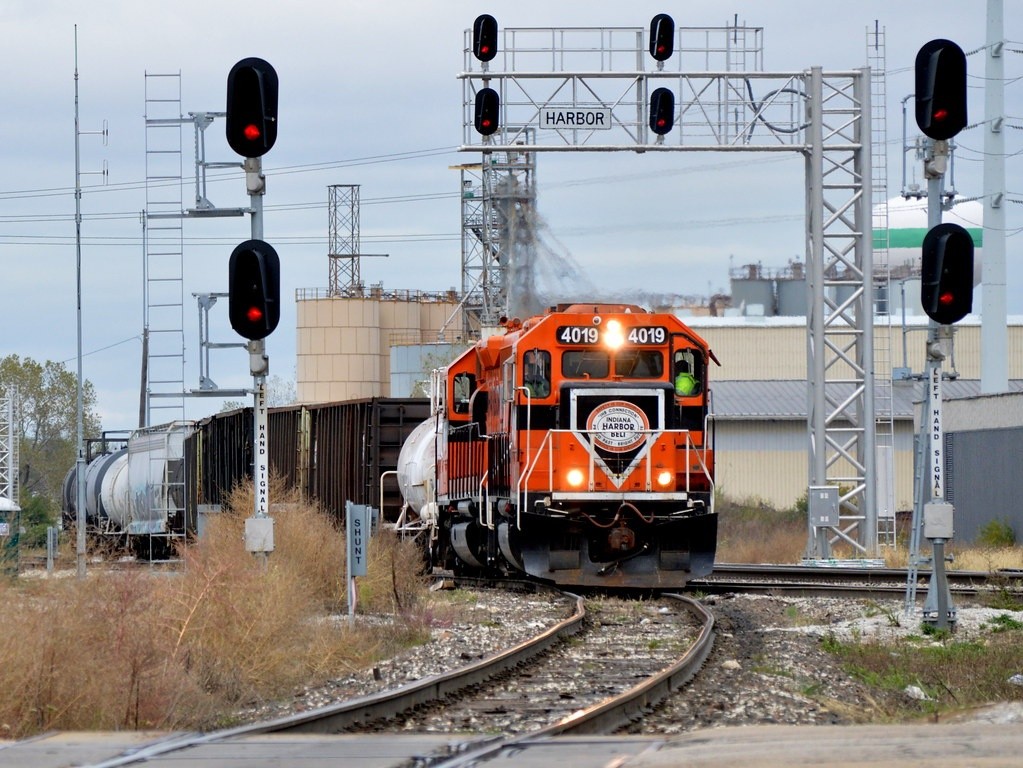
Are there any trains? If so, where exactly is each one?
[59,298,723,594]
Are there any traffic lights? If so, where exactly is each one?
[649,14,675,62]
[228,239,281,340]
[650,86,674,134]
[921,223,976,325]
[225,57,279,157]
[472,14,497,61]
[915,39,968,140]
[474,88,499,136]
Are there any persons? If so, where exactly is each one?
[675,359,701,398]
[525,362,550,397]
